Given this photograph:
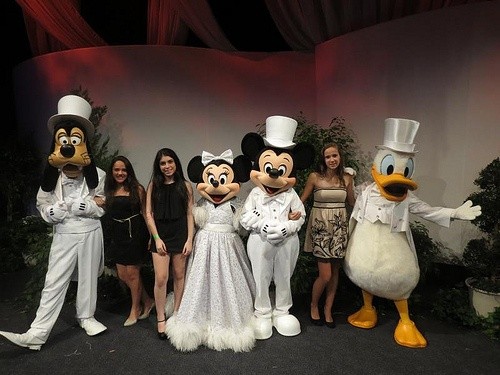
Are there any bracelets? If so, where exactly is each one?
[152,234,159,239]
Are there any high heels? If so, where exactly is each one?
[139,300,155,319]
[321,307,336,328]
[124,306,143,327]
[309,304,322,327]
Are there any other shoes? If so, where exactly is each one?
[157,317,167,339]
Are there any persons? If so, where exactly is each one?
[146,147,194,340]
[287,142,358,328]
[94,154,154,326]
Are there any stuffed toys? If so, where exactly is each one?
[341,116,483,347]
[167,148,255,352]
[241,116,307,339]
[0,95,108,351]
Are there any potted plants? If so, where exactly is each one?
[463,157,500,321]
